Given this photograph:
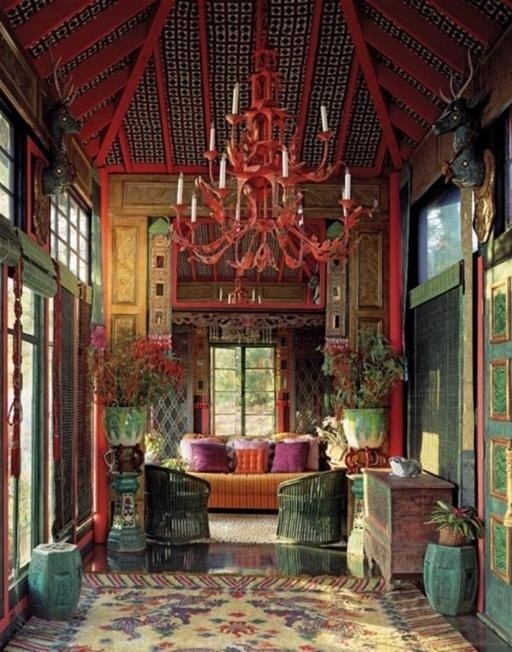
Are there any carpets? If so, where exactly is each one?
[146,513,347,547]
[1,572,476,652]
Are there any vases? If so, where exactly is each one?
[101,405,147,447]
[342,409,385,451]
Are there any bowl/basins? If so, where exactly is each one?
[389,460,417,477]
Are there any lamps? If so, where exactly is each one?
[219,277,262,328]
[169,16,377,276]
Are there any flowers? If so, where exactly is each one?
[315,330,406,408]
[315,416,347,447]
[91,326,183,407]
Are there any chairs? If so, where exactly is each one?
[276,468,346,544]
[142,462,210,542]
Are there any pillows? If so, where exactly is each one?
[232,441,269,470]
[191,444,229,473]
[235,447,266,474]
[284,438,320,470]
[271,441,309,473]
[186,437,220,471]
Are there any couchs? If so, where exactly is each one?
[181,434,321,509]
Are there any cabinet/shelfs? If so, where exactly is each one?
[361,468,455,600]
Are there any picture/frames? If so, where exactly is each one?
[489,360,511,421]
[489,275,511,344]
[490,513,511,586]
[489,437,511,501]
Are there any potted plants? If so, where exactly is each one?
[426,500,484,547]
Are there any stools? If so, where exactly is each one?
[424,543,478,616]
[28,543,82,620]
